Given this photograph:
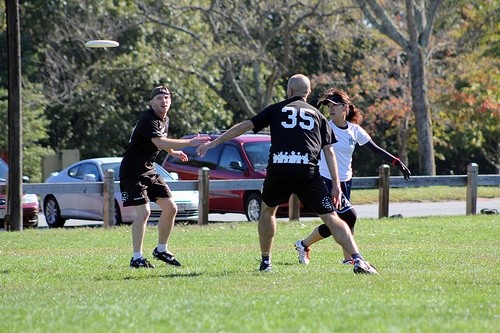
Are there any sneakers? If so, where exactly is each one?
[258,260,272,272]
[130,257,156,270]
[354,257,379,275]
[342,258,354,265]
[152,247,182,268]
[294,240,310,265]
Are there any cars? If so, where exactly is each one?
[0,160,40,228]
[39,157,200,226]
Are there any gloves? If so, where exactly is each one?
[395,159,412,182]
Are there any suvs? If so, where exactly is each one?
[160,131,323,217]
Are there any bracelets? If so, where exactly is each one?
[392,158,400,165]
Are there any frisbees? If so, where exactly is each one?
[85,40,120,48]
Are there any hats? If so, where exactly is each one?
[322,97,346,105]
[150,86,169,99]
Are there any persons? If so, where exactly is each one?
[119,86,211,267]
[294,91,412,266]
[193,74,378,274]
